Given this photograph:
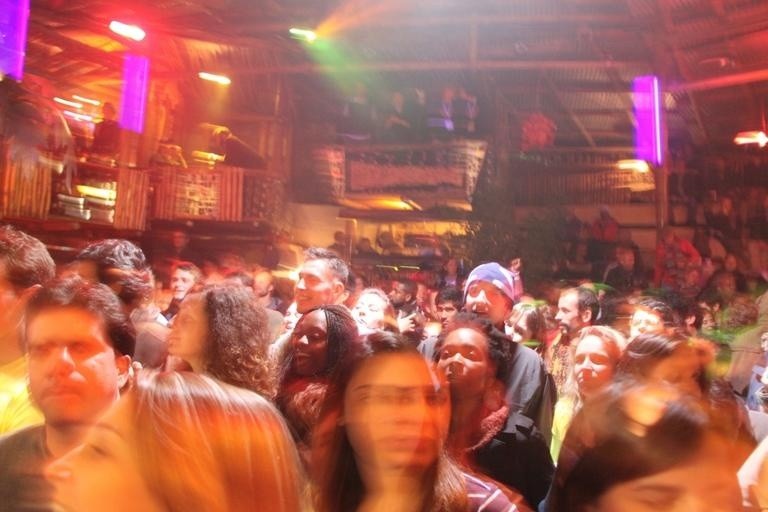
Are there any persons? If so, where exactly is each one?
[91,103,121,158]
[2,156,768,512]
[342,79,487,139]
[212,126,267,169]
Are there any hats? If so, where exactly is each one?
[463,261,518,302]
